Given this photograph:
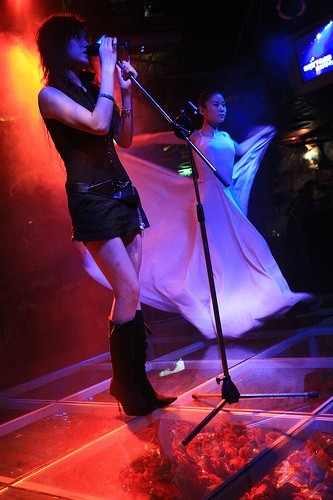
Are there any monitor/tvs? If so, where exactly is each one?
[293,16,333,85]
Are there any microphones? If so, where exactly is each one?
[87,39,128,56]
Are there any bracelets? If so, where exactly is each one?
[121,107,131,113]
[98,94,114,102]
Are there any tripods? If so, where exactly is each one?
[116,59,320,447]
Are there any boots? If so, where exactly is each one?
[108,310,177,416]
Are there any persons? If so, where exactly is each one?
[37,15,179,418]
[72,91,312,340]
